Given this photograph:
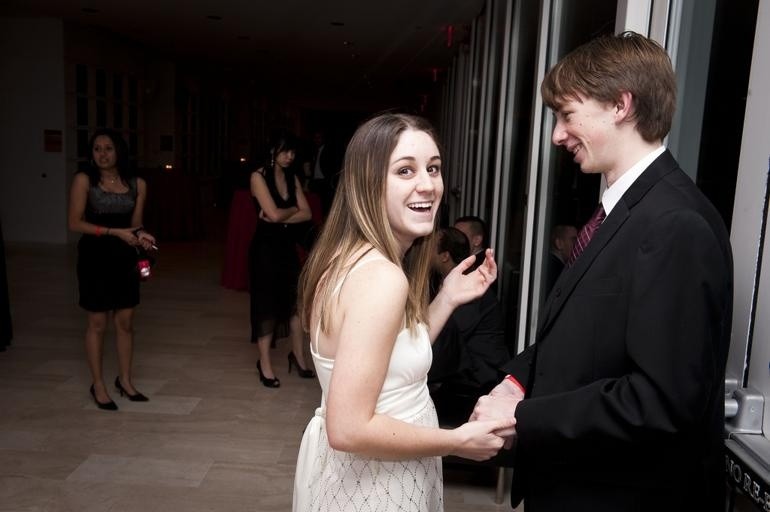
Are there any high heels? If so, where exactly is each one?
[89,381,118,411]
[114,374,150,402]
[256,358,280,389]
[287,350,317,379]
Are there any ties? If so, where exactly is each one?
[566,202,606,272]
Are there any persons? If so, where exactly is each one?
[544,224,579,300]
[464,27,737,512]
[424,227,513,487]
[245,121,317,388]
[452,216,498,293]
[67,128,159,413]
[307,133,340,223]
[288,110,520,511]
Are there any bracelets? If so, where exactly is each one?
[504,373,527,395]
[133,225,144,238]
[94,225,115,239]
